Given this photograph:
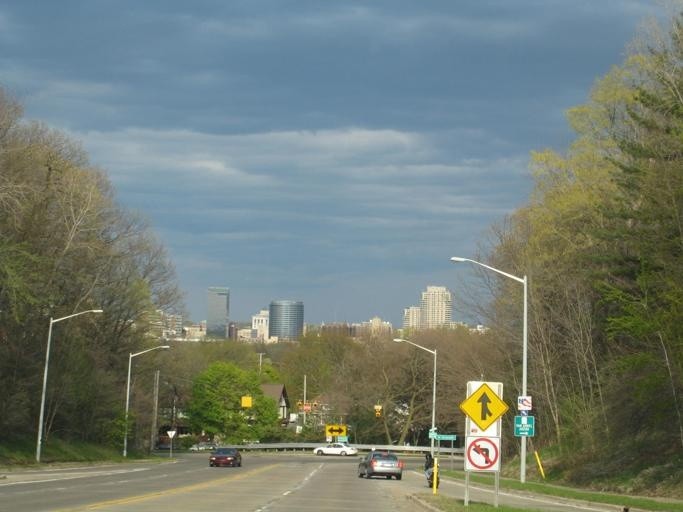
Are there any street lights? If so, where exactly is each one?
[450,256,527,483]
[123,345,170,457]
[393,338,437,458]
[36,309,103,462]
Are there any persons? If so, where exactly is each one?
[423,453,440,479]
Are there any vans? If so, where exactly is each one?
[159,424,192,446]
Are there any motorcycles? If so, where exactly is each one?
[427,472,439,488]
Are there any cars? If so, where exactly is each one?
[359,449,402,480]
[313,443,358,456]
[209,448,241,467]
